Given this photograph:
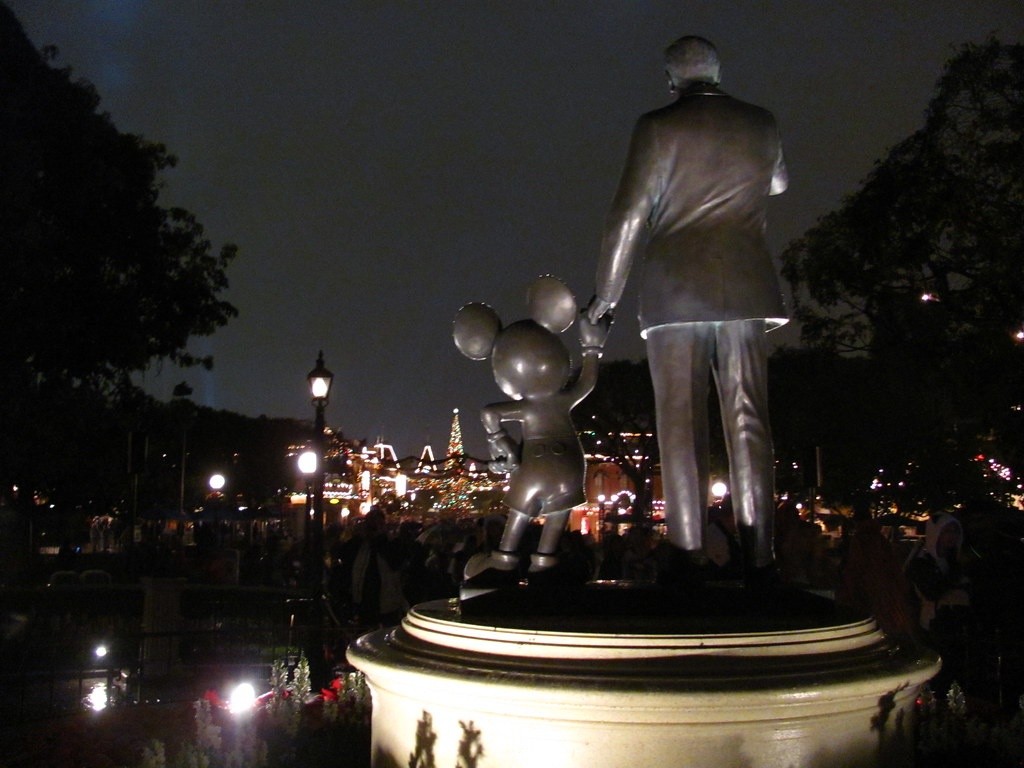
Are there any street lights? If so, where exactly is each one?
[305,355,334,558]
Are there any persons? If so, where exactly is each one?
[193,520,226,544]
[90,516,123,552]
[330,496,1024,722]
[266,521,276,538]
[588,36,791,597]
[140,519,160,537]
[253,522,259,540]
[177,521,184,537]
